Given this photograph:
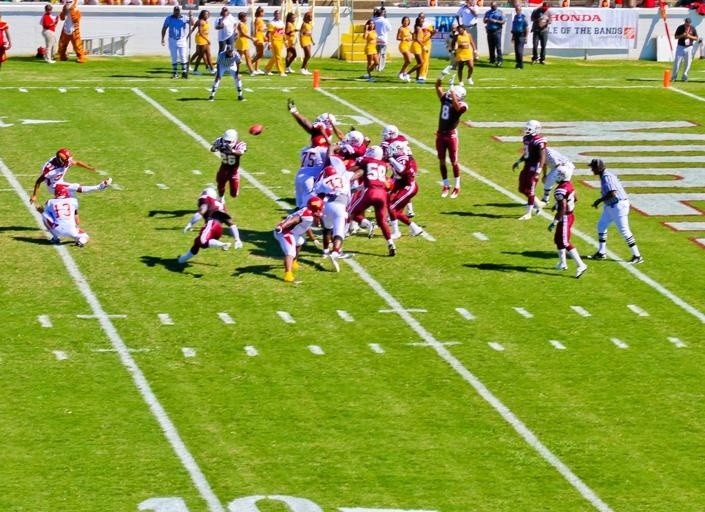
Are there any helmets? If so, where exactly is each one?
[446,85,467,104]
[391,142,409,159]
[45,5,53,11]
[346,131,362,148]
[383,125,398,140]
[222,128,238,150]
[53,184,71,198]
[313,135,326,147]
[320,166,337,179]
[199,188,219,202]
[523,120,543,136]
[306,196,325,216]
[56,148,72,169]
[554,166,573,184]
[316,112,336,132]
[366,145,384,160]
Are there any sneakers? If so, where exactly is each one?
[248,67,312,78]
[47,236,60,245]
[362,71,428,84]
[626,255,644,265]
[169,66,218,80]
[98,177,113,191]
[488,58,545,70]
[221,241,232,252]
[587,251,608,260]
[208,95,214,101]
[575,263,588,279]
[237,96,248,102]
[439,185,461,199]
[176,254,185,267]
[460,78,474,86]
[345,208,423,256]
[554,262,568,271]
[291,258,301,272]
[37,54,86,65]
[519,201,551,223]
[321,248,342,272]
[283,271,295,283]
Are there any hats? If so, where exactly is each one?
[586,159,606,173]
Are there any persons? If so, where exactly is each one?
[159,3,567,85]
[40,5,59,64]
[670,18,699,82]
[207,127,246,200]
[205,44,249,106]
[512,118,579,221]
[0,19,12,72]
[35,184,90,246]
[549,167,588,278]
[587,156,645,267]
[29,147,113,205]
[431,65,473,200]
[175,186,244,267]
[269,97,437,283]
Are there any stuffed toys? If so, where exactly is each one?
[58,0,85,63]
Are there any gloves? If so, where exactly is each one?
[552,205,557,213]
[548,223,555,233]
[230,224,243,251]
[329,114,339,126]
[438,65,456,85]
[273,226,282,236]
[183,212,203,234]
[311,240,321,248]
[286,98,296,112]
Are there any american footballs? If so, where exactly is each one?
[249,125,263,135]
